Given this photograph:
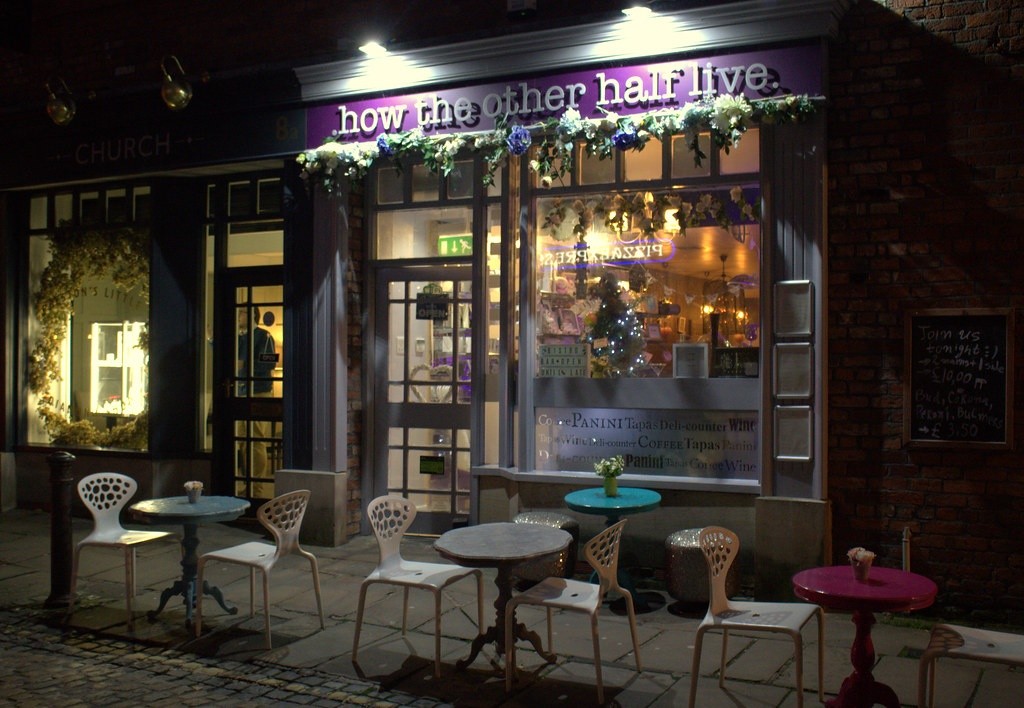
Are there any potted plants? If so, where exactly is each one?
[595,454,625,497]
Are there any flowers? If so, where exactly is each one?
[847,548,876,566]
[183,480,205,491]
[285,91,778,237]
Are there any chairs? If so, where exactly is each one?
[500,518,643,703]
[686,527,827,708]
[352,495,484,679]
[196,489,325,649]
[68,472,185,630]
[916,623,1024,708]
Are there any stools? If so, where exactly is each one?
[510,512,579,584]
[666,529,746,604]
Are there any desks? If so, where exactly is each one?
[129,496,251,622]
[564,487,661,606]
[435,523,573,682]
[793,566,937,708]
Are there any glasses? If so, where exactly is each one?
[239,310,247,314]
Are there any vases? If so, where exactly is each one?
[851,560,872,581]
[188,491,203,503]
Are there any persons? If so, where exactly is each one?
[237,302,277,498]
